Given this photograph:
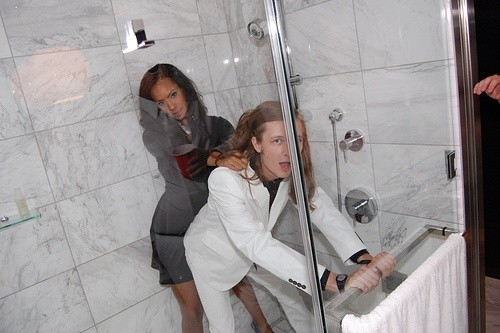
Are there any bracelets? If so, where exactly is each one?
[215,153,223,167]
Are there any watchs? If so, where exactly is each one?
[336,274,348,293]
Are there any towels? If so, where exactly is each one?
[341,232,469,333]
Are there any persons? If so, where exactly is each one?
[138,63,276,333]
[182,100,397,333]
[474,74,500,103]
